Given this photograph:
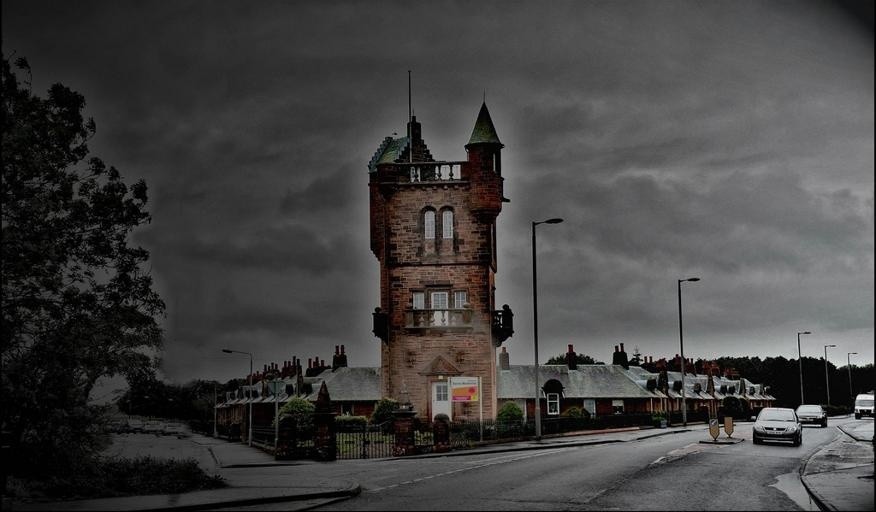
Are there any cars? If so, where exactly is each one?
[753,406,802,448]
[795,404,828,427]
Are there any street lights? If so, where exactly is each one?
[221,349,253,448]
[677,278,700,425]
[798,332,812,405]
[531,218,564,440]
[824,345,836,407]
[848,353,857,399]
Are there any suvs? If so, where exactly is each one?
[854,394,875,419]
